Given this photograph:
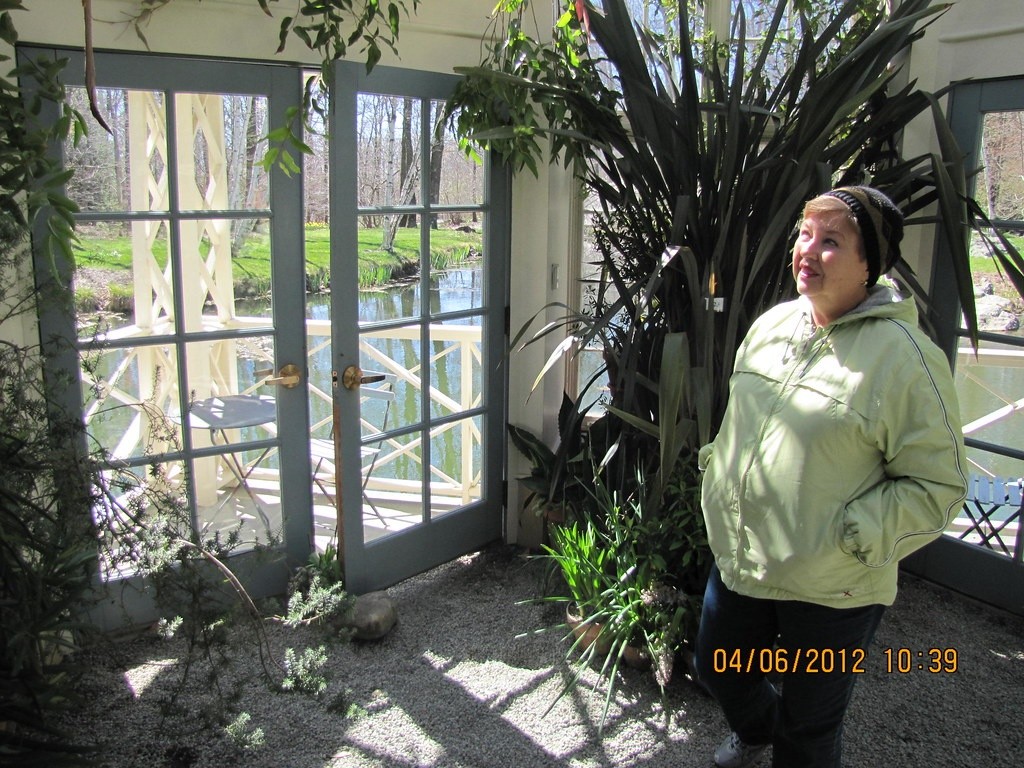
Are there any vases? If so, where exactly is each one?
[616,638,653,671]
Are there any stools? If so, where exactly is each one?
[958,472,1024,559]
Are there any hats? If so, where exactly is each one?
[826,184,904,288]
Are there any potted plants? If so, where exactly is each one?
[506,390,622,551]
[539,520,621,657]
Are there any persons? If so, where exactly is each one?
[695,187,968,768]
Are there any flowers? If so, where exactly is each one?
[540,577,695,738]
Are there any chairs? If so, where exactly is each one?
[275,369,398,540]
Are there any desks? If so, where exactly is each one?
[169,394,278,540]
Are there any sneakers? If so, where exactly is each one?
[713,731,770,768]
